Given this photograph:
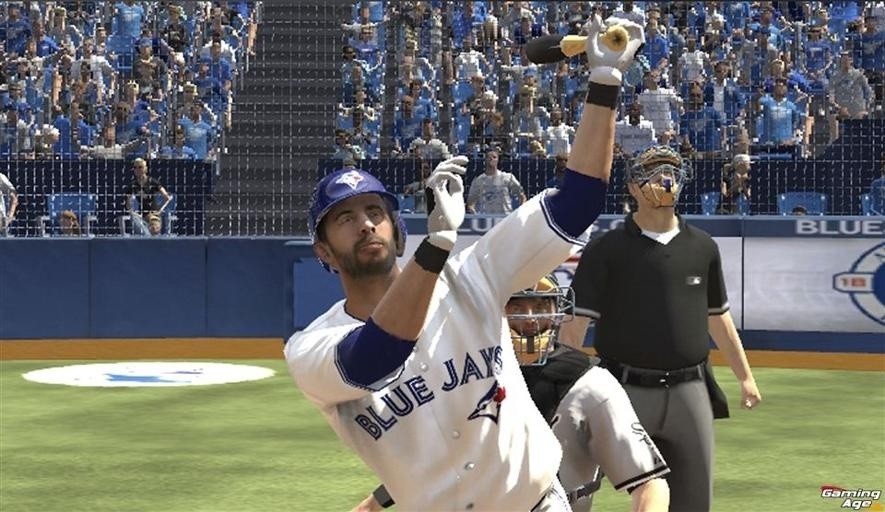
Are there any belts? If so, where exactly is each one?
[617,369,701,391]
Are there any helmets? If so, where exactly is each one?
[625,145,688,208]
[505,272,574,368]
[306,167,405,273]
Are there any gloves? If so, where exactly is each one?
[585,14,644,111]
[416,154,470,274]
